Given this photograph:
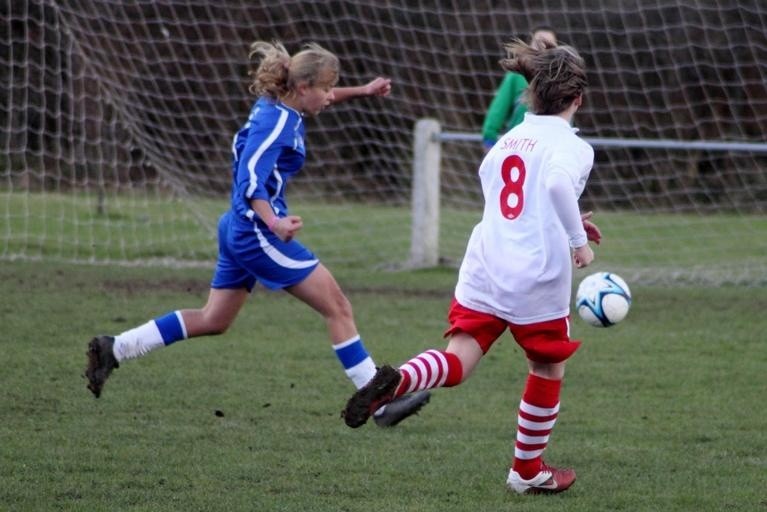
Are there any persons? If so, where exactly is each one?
[480,30,556,153]
[87,41,430,428]
[347,43,601,495]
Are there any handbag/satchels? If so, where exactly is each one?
[266,216,278,232]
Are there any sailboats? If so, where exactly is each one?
[576,271,631,328]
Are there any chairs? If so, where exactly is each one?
[373,391,430,428]
[345,366,401,428]
[506,461,576,495]
[86,336,119,398]
